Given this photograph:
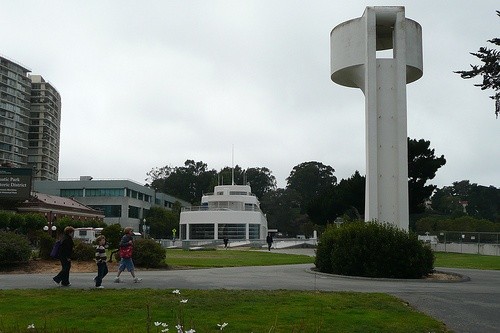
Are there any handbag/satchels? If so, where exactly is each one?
[120,246,133,258]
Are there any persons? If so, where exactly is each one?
[223,236,229,248]
[92,235,108,288]
[52,226,74,287]
[266,233,273,252]
[115,227,142,282]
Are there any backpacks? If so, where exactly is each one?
[50,240,62,257]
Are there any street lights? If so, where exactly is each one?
[142,218,147,239]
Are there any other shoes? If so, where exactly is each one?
[53,280,60,286]
[113,277,120,282]
[133,277,142,282]
[61,282,71,286]
[92,278,97,283]
[95,285,104,288]
